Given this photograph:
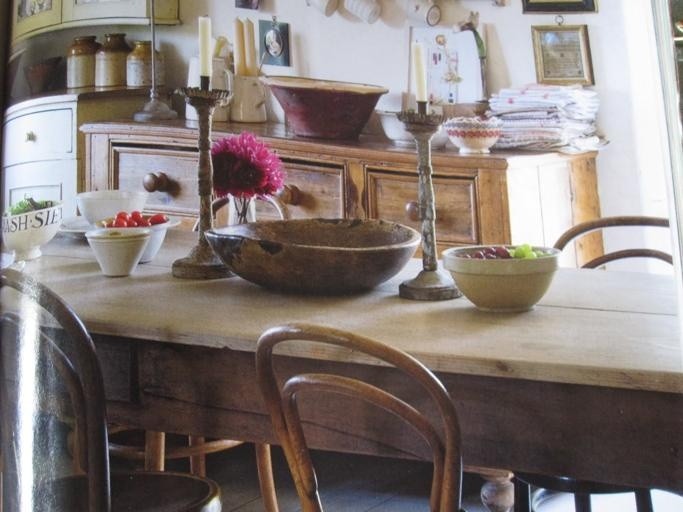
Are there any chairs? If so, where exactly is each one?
[506,215,676,508]
[253,323,465,512]
[1,267,220,510]
[110,186,287,512]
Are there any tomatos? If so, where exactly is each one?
[139,219,149,227]
[148,214,166,223]
[131,211,141,219]
[112,218,127,227]
[115,211,127,221]
[125,220,136,227]
[106,219,114,227]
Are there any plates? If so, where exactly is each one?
[58,215,183,242]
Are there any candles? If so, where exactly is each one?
[244,14,257,79]
[414,37,429,103]
[234,14,245,76]
[198,12,213,78]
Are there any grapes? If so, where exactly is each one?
[451,245,554,258]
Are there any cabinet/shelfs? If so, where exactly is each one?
[7,1,184,48]
[0,87,188,232]
[76,114,609,274]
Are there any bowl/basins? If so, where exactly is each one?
[441,245,562,313]
[0,200,64,262]
[72,189,148,226]
[375,108,450,149]
[94,214,171,265]
[88,228,152,278]
[202,217,422,296]
[443,121,502,159]
[258,73,389,143]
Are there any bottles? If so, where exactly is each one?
[126,40,164,86]
[66,35,101,89]
[94,32,129,86]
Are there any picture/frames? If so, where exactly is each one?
[530,23,595,88]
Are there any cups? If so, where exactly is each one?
[232,72,268,124]
[184,55,232,122]
